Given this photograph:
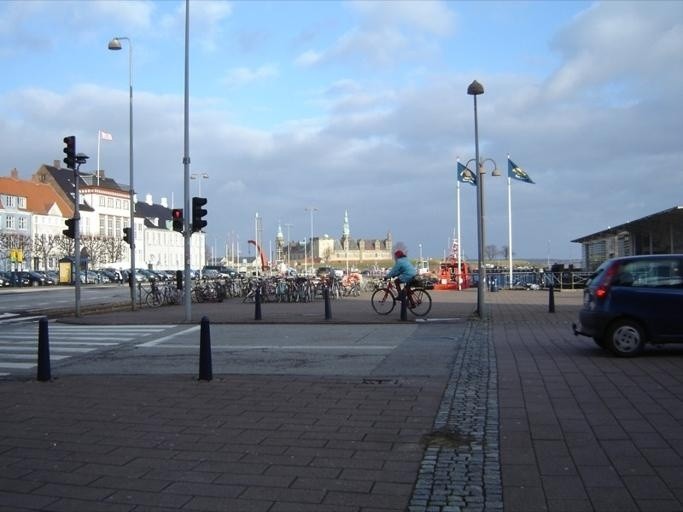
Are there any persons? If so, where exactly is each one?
[380,248,419,309]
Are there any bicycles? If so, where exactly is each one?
[143,272,364,309]
[372,277,434,317]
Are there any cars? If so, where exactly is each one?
[0,265,245,289]
[571,252,683,356]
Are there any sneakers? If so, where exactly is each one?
[395,295,402,301]
[409,305,416,309]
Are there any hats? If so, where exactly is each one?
[394,250,406,258]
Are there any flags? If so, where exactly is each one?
[457,161,476,187]
[507,157,535,185]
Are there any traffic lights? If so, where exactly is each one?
[191,197,208,234]
[60,217,76,238]
[171,208,183,232]
[122,227,133,246]
[62,134,75,170]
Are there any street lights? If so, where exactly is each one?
[461,156,500,318]
[189,170,210,280]
[107,36,136,311]
[304,207,318,274]
[284,224,293,271]
